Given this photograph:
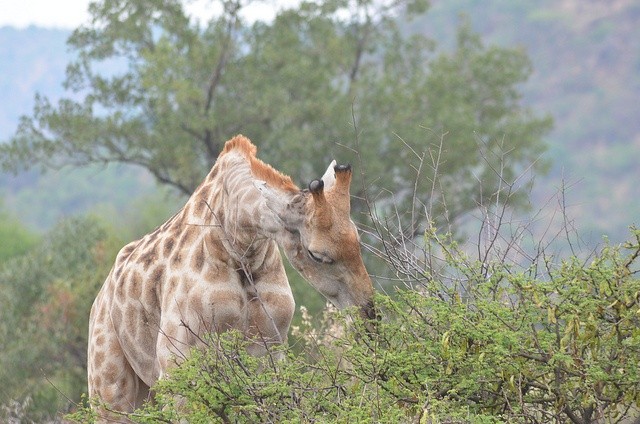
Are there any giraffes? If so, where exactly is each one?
[87,135,381,424]
